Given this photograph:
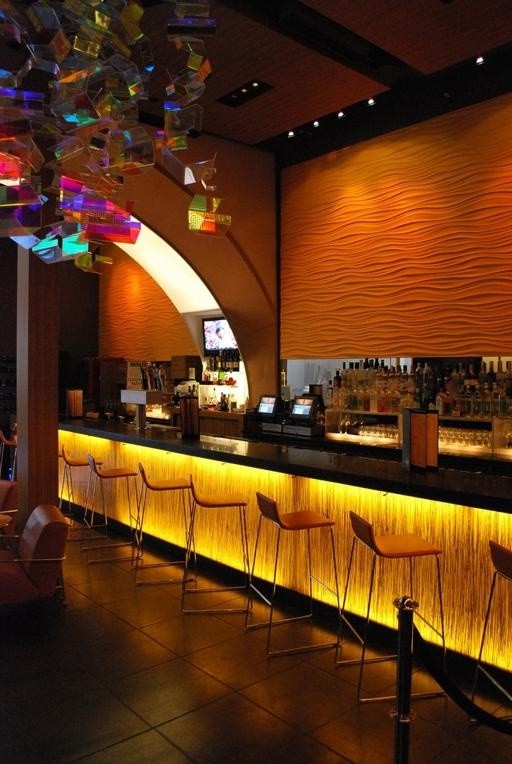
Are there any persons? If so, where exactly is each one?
[141,360,167,393]
[215,328,236,348]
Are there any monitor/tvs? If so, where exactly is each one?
[289,396,317,420]
[256,395,278,418]
[201,316,241,357]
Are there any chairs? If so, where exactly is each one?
[0,503,69,618]
[0,430,17,480]
[0,480,21,534]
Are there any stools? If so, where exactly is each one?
[132,464,198,585]
[60,445,109,530]
[245,492,342,657]
[180,475,253,615]
[334,512,446,704]
[80,454,143,565]
[471,538,511,722]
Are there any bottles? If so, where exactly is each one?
[203,348,240,381]
[218,392,231,412]
[188,385,199,396]
[328,357,512,418]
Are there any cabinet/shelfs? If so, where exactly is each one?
[170,410,243,437]
[325,408,403,450]
[438,415,512,464]
